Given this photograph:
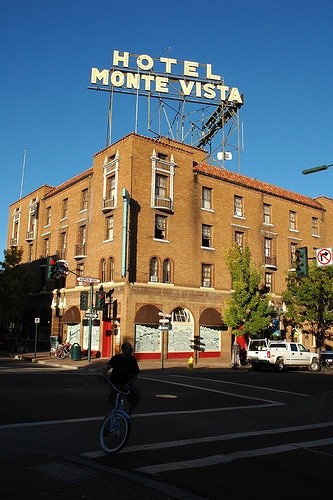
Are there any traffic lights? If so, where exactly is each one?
[44,255,57,282]
[295,246,308,278]
[80,291,88,310]
[95,291,106,310]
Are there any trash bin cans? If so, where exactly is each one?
[71,343,81,360]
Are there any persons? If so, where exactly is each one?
[239,347,246,365]
[104,343,142,416]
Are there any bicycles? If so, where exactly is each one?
[54,341,72,360]
[98,376,134,453]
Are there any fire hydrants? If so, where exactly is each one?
[188,356,195,370]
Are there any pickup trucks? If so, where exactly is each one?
[245,337,320,374]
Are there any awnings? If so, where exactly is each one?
[199,308,226,327]
[60,306,81,323]
[134,304,163,325]
